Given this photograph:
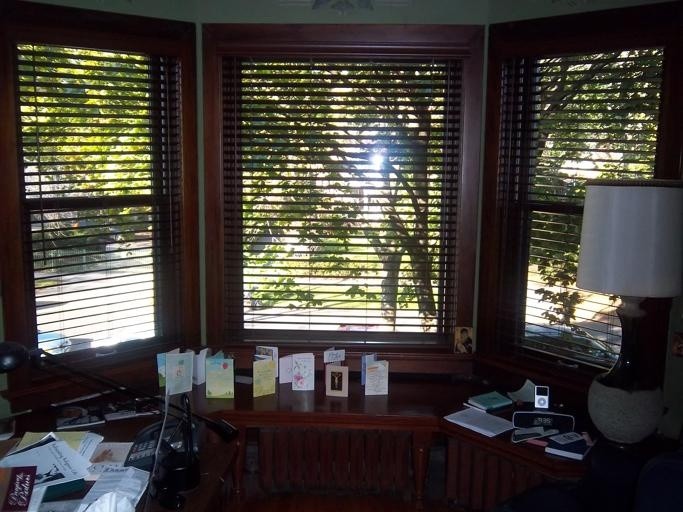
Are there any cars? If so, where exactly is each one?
[524,319,618,361]
[36,329,73,358]
[46,220,123,252]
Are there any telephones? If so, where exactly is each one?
[124,418,201,490]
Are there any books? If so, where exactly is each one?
[462,399,509,414]
[465,390,513,411]
[0,430,136,511]
[544,430,597,462]
[154,344,390,399]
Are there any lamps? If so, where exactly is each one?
[0,340,241,494]
[572,181,682,446]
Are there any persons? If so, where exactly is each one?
[454,327,472,353]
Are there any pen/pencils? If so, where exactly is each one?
[506,391,522,406]
[463,403,486,412]
